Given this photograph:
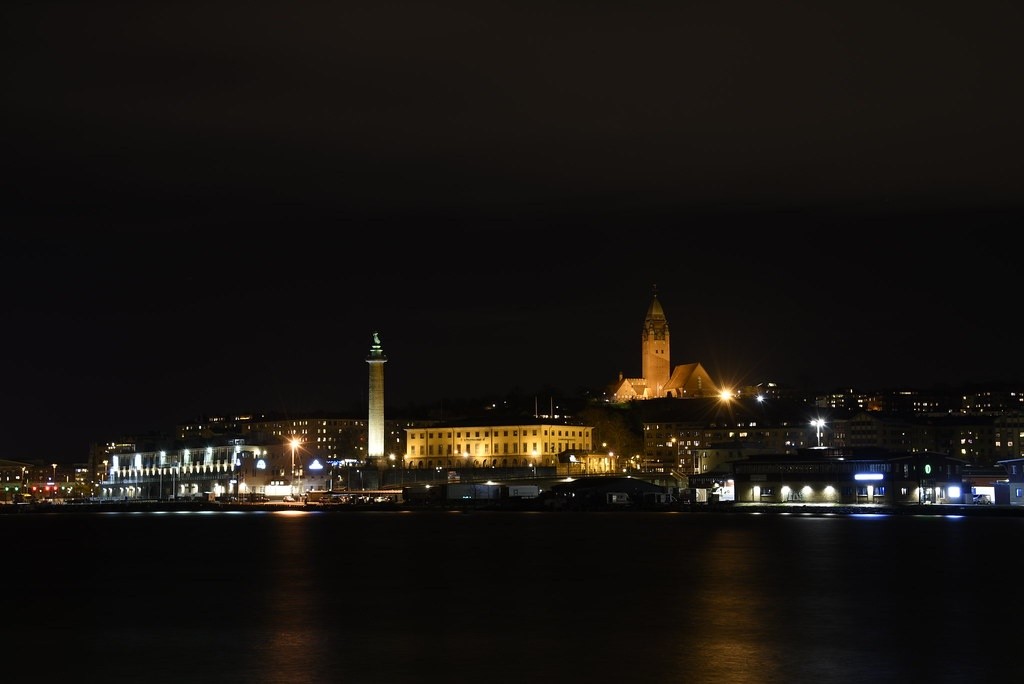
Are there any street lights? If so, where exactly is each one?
[290,439,300,497]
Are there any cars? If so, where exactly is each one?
[283,496,294,503]
